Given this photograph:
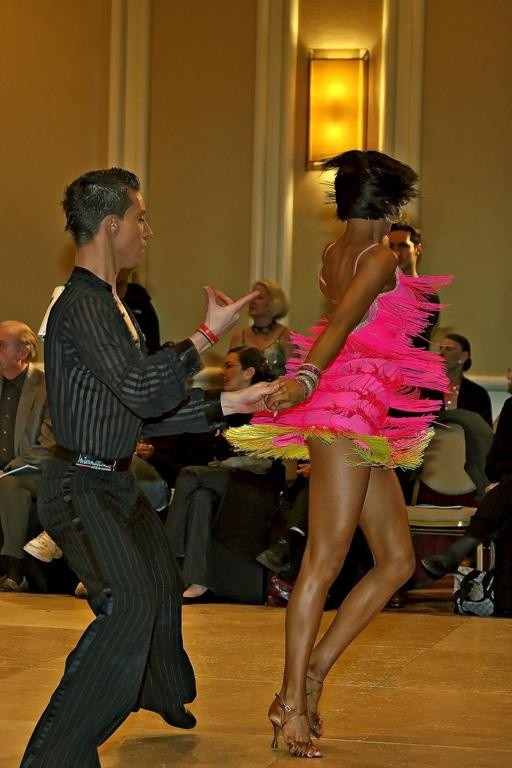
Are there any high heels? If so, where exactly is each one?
[268,675,324,758]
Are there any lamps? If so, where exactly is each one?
[306,48,369,171]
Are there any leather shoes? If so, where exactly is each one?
[141,704,196,729]
[421,553,458,579]
[181,588,216,604]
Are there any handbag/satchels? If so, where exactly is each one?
[452,566,494,616]
[267,572,336,611]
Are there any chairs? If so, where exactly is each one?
[406,424,495,599]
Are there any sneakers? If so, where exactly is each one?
[75,582,89,599]
[0,574,29,592]
[255,537,291,573]
[23,529,63,563]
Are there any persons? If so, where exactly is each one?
[267,148,457,760]
[22,169,279,766]
[0,321,512,613]
[37,242,80,346]
[229,278,301,374]
[115,265,162,357]
